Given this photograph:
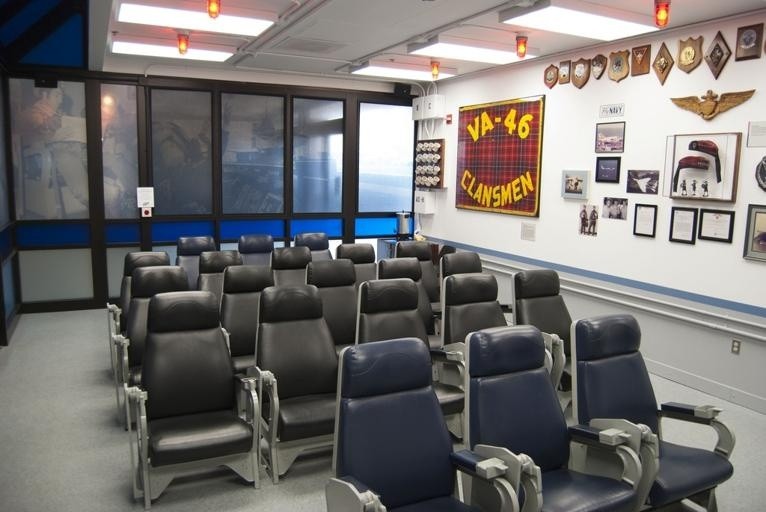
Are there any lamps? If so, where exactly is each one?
[431,60,440,76]
[407,35,540,68]
[350,58,458,83]
[498,0,661,44]
[516,36,526,58]
[176,32,189,53]
[207,0,220,18]
[102,93,115,112]
[655,1,671,26]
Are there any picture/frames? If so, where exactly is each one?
[626,169,659,194]
[544,63,558,89]
[560,170,589,200]
[579,202,600,236]
[560,60,570,85]
[595,121,626,154]
[653,41,675,86]
[669,206,698,245]
[592,54,607,79]
[698,208,720,241]
[678,36,702,75]
[595,156,620,183]
[668,133,720,201]
[747,120,765,148]
[703,30,733,81]
[571,57,591,90]
[633,203,659,238]
[609,51,630,82]
[630,45,651,76]
[742,204,765,263]
[602,197,628,219]
[755,156,766,189]
[734,22,764,62]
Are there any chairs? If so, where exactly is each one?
[303,259,360,357]
[571,314,736,512]
[463,324,643,512]
[337,243,377,286]
[176,235,217,290]
[248,284,343,484]
[270,247,312,287]
[511,269,572,391]
[355,278,466,445]
[238,235,274,267]
[378,257,442,351]
[111,265,238,423]
[122,290,261,510]
[198,250,243,302]
[441,272,565,407]
[440,253,482,278]
[395,240,439,314]
[106,251,172,376]
[294,232,331,261]
[221,265,277,373]
[442,273,551,401]
[324,337,520,512]
